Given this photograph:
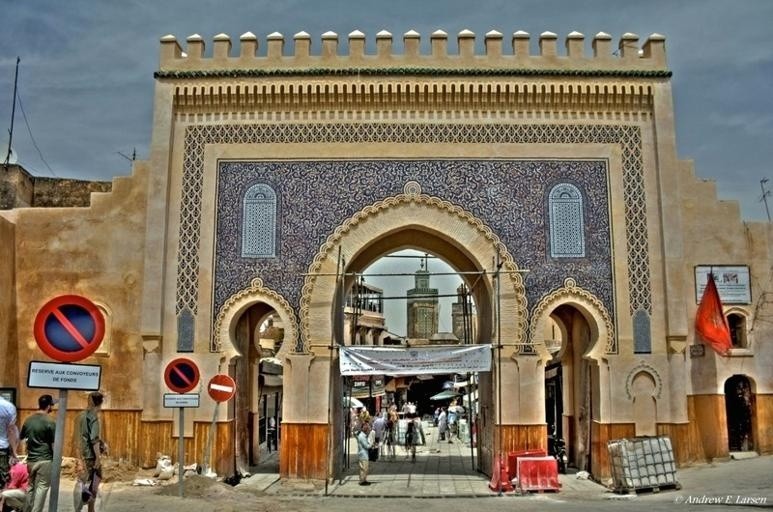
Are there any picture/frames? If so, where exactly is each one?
[694,264,752,306]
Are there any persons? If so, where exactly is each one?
[0,397,19,512]
[345,402,454,486]
[74,392,103,512]
[9,394,55,512]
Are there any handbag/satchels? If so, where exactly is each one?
[369,447,378,462]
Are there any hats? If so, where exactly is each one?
[39,395,59,405]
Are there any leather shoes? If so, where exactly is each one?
[360,481,371,485]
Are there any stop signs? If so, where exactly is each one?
[208,375,236,403]
[164,358,200,393]
[34,295,105,363]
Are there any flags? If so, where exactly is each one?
[695,273,734,357]
